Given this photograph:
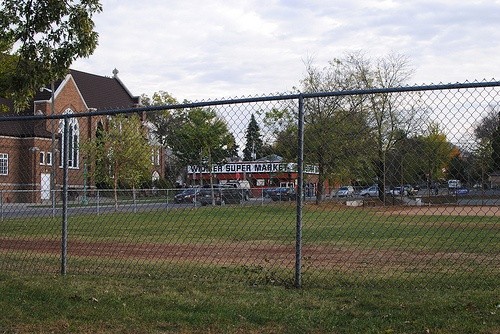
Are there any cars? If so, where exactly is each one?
[173,179,493,207]
